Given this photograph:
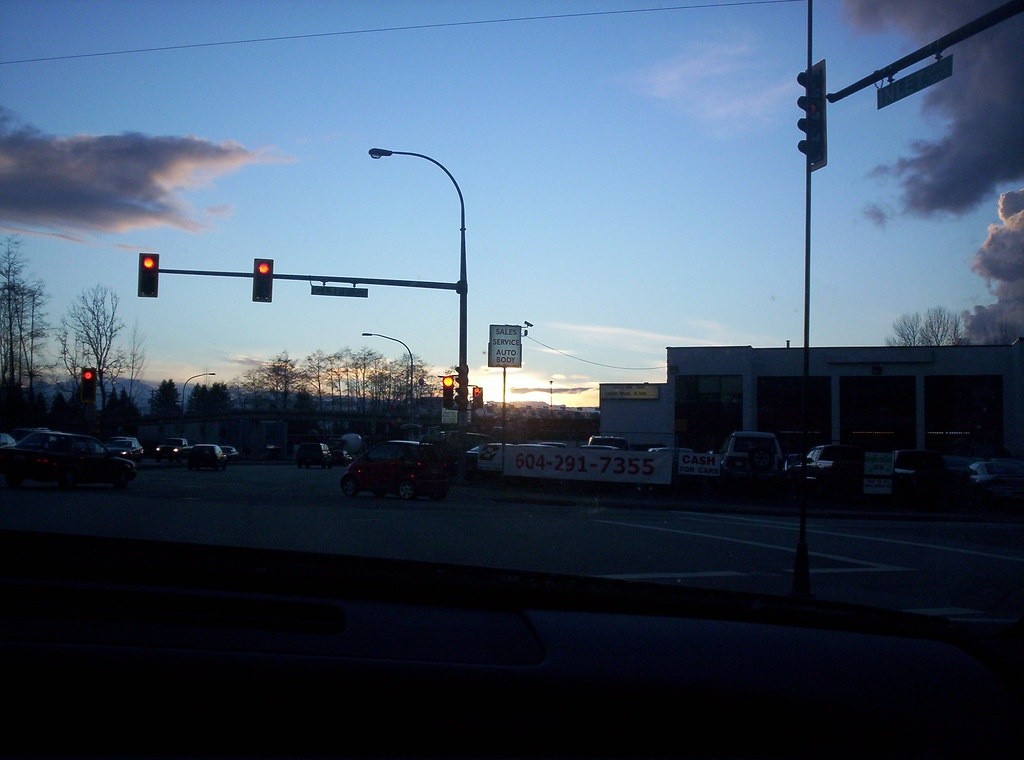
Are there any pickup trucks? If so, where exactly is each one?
[154,438,194,463]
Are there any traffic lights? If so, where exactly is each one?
[252,258,273,303]
[474,388,483,400]
[455,364,469,407]
[80,367,97,403]
[442,376,454,410]
[138,253,159,298]
[796,59,827,173]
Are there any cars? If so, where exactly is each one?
[189,444,239,471]
[0,428,144,491]
[785,444,1023,499]
[340,440,450,501]
[421,432,727,496]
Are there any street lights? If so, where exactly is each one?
[369,148,468,428]
[549,381,553,407]
[180,373,216,438]
[362,333,413,422]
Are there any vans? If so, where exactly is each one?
[296,442,333,469]
[720,431,784,477]
